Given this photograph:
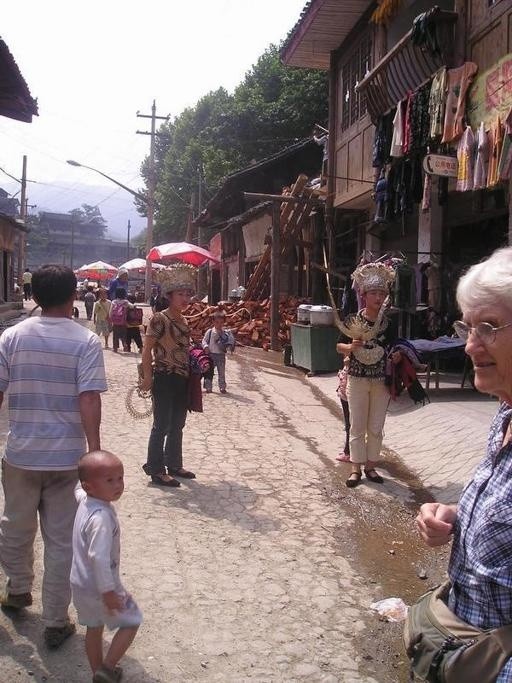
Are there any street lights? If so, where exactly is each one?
[63,157,156,306]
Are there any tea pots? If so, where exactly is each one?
[232,285,245,295]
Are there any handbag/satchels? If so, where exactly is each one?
[403,582,510,682]
[186,374,202,412]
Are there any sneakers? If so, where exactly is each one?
[336,453,349,461]
[346,472,362,487]
[94,663,118,682]
[152,474,180,486]
[115,666,122,677]
[168,469,195,478]
[363,469,383,482]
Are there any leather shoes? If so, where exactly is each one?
[45,623,75,649]
[1,593,32,607]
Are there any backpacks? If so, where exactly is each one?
[111,301,127,326]
[126,308,143,325]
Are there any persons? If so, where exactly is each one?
[76,277,169,353]
[201,313,235,394]
[335,262,404,490]
[138,263,210,488]
[22,268,32,301]
[417,244,511,681]
[70,447,144,682]
[0,265,108,647]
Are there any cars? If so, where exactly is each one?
[14,277,20,293]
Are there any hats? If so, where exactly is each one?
[351,263,397,296]
[153,264,199,298]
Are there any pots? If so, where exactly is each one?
[297,304,312,323]
[310,305,337,327]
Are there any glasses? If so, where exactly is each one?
[452,320,510,345]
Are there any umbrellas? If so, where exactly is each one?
[74,262,88,277]
[119,255,166,279]
[148,241,219,267]
[77,260,119,287]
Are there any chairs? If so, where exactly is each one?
[416,350,443,393]
